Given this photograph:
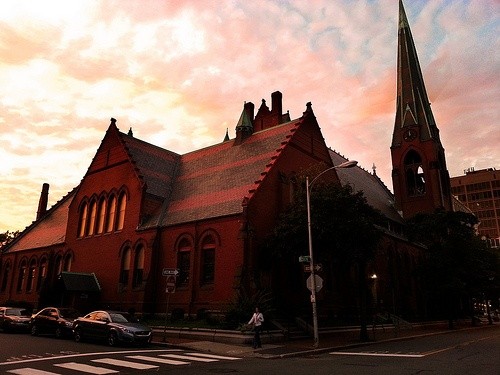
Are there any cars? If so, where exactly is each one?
[31,307,86,339]
[72,310,153,348]
[0,307,31,333]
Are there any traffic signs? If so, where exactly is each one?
[162,267,180,276]
[304,264,323,273]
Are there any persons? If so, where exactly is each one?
[248,307,264,349]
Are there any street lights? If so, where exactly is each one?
[304,158,359,348]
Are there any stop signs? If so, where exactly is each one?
[166,274,176,294]
